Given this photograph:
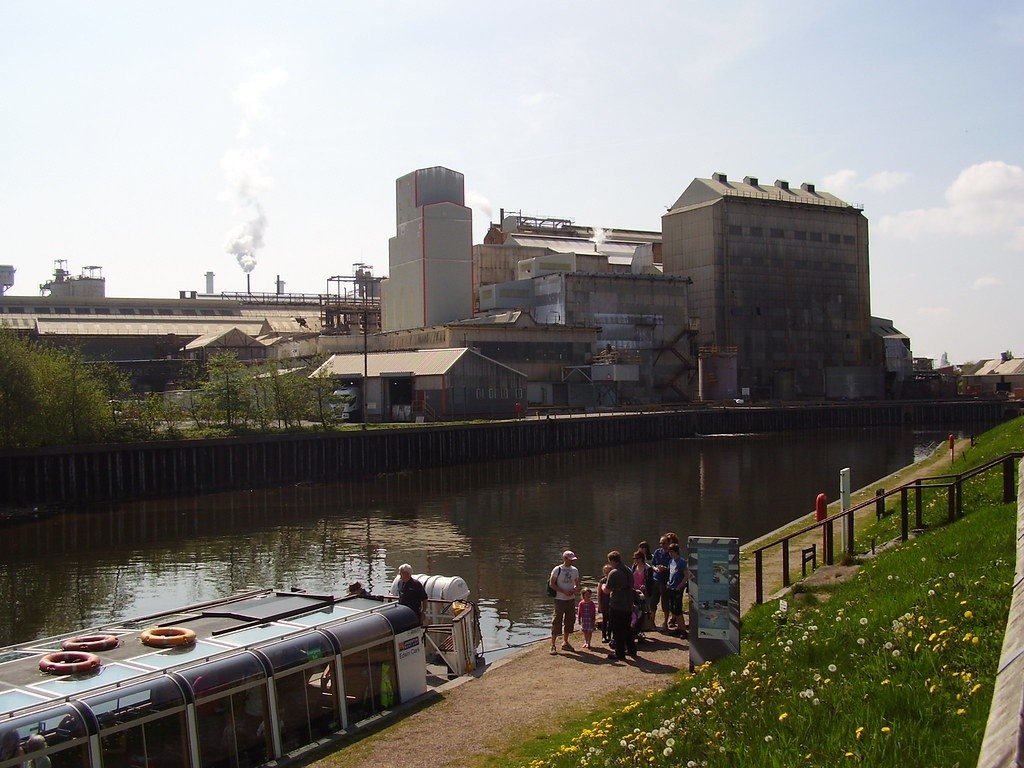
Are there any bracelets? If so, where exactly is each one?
[423,611,426,614]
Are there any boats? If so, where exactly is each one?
[1,590,432,768]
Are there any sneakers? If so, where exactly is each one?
[562,643,574,651]
[549,644,557,655]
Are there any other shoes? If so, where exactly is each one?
[656,622,668,630]
[609,653,624,658]
[602,636,611,642]
[625,650,637,656]
[582,642,590,648]
[672,629,686,634]
[669,618,677,627]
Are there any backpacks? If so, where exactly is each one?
[641,610,653,631]
[546,567,561,597]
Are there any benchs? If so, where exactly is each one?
[435,636,456,672]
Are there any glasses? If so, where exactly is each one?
[633,557,641,559]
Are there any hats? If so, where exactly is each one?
[639,541,650,548]
[347,581,363,592]
[562,550,577,561]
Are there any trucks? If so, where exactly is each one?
[330,388,363,421]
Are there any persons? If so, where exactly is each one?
[578,587,596,648]
[398,563,428,626]
[638,541,654,563]
[665,543,688,638]
[101,712,139,768]
[666,533,683,557]
[549,549,581,654]
[598,551,637,659]
[650,536,672,632]
[57,715,81,741]
[256,704,285,737]
[631,549,653,632]
[597,565,613,643]
[223,712,249,768]
[29,735,52,768]
[0,730,32,768]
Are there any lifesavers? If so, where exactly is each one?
[62,634,119,652]
[140,627,197,648]
[38,651,101,675]
